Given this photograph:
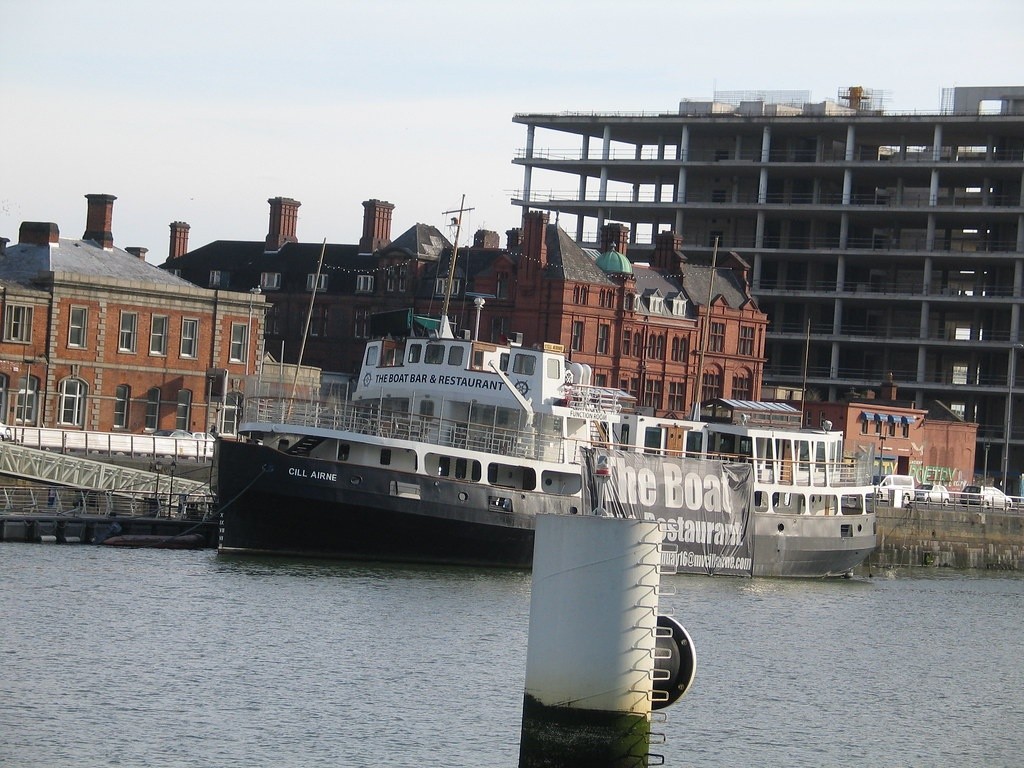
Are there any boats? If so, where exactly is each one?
[203,191,881,579]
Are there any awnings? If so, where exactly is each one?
[889,415,902,423]
[902,416,915,424]
[875,413,888,422]
[861,411,875,420]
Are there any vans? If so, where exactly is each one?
[873,474,915,503]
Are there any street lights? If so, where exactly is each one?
[244,285,262,419]
[878,436,886,483]
[981,444,991,512]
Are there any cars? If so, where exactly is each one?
[916,483,950,507]
[958,486,1013,510]
[0,421,13,441]
